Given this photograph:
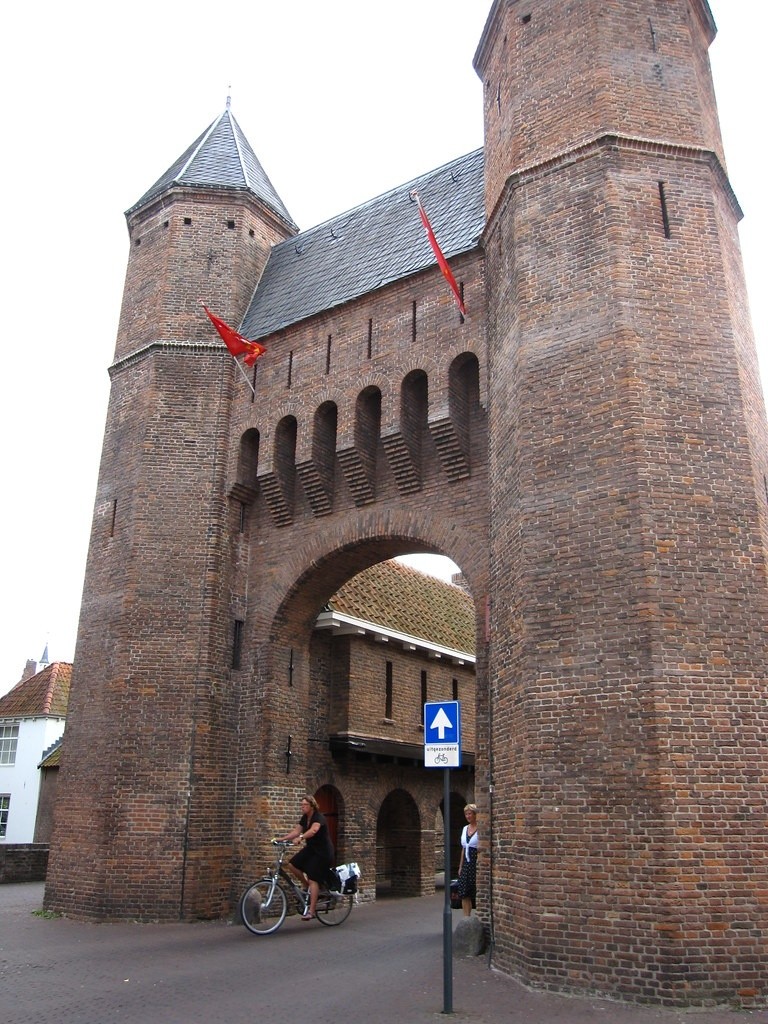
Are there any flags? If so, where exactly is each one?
[419,204,466,315]
[202,304,267,367]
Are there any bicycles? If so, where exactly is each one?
[237,837,356,936]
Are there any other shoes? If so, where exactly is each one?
[302,912,316,920]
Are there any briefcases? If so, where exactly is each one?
[450,880,476,909]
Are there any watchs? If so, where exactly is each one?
[299,834,304,840]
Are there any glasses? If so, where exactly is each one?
[301,803,309,806]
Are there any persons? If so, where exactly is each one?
[459,804,478,916]
[271,794,334,920]
